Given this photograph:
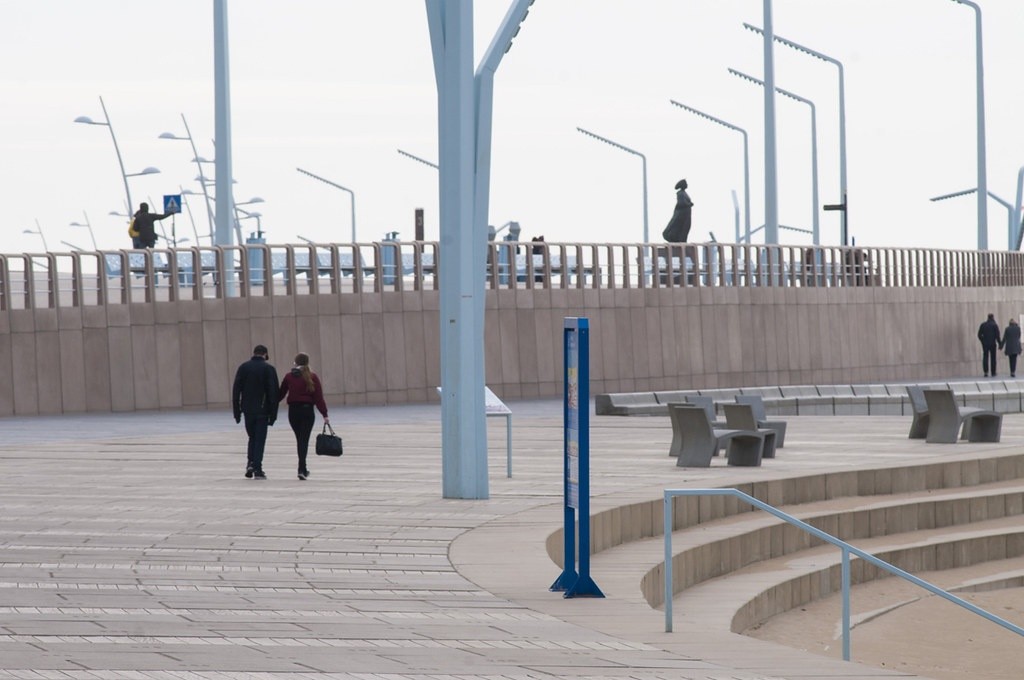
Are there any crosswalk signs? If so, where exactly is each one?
[163,195,183,213]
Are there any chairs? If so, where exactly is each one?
[906,384,1004,442]
[668,395,787,468]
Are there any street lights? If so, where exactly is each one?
[742,22,848,245]
[728,66,819,244]
[668,98,751,247]
[293,167,358,244]
[576,126,651,256]
[21,94,270,252]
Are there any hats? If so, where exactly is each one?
[254,345,269,360]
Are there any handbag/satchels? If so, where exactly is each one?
[315,422,342,456]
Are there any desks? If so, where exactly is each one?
[437,387,513,478]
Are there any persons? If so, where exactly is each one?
[800,247,815,288]
[274,352,330,480]
[978,313,1002,377]
[998,318,1022,378]
[661,180,694,242]
[232,344,279,480]
[128,202,174,249]
[531,236,550,282]
[839,245,869,287]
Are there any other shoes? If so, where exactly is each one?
[297,472,306,480]
[245,463,253,478]
[305,471,309,476]
[255,473,266,479]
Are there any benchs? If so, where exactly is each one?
[268,250,374,286]
[402,252,434,279]
[595,379,1024,416]
[106,252,219,286]
[514,254,602,287]
[637,257,693,281]
